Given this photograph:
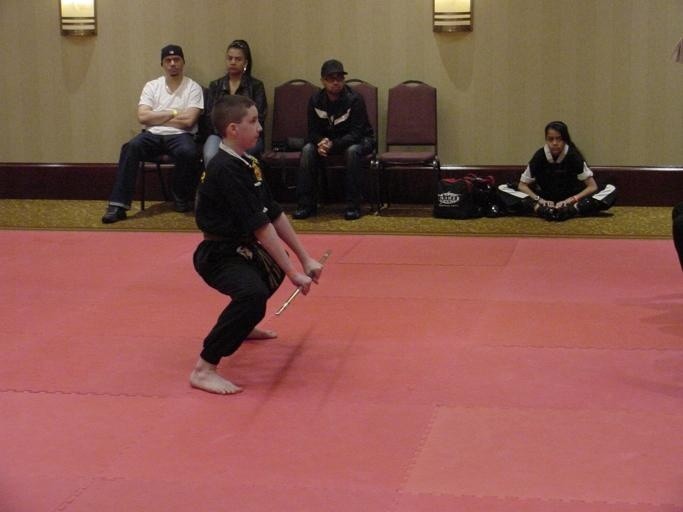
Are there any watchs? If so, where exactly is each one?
[533,195,540,202]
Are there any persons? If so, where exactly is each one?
[498,119,617,222]
[191,94,323,395]
[291,59,379,222]
[198,38,267,170]
[102,45,207,224]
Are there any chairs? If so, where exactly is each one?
[378,78,440,214]
[319,79,378,212]
[262,77,325,210]
[137,86,208,209]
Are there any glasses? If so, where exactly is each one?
[326,75,344,82]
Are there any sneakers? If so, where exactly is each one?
[345,206,359,219]
[292,206,318,218]
[102,206,127,225]
[172,187,190,212]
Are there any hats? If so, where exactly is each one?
[161,44,185,66]
[321,60,348,75]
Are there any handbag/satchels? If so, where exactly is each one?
[496,181,534,215]
[433,173,495,217]
[272,136,304,153]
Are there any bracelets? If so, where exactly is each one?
[171,109,178,117]
[572,194,579,203]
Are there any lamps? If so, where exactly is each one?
[432,0,474,34]
[58,1,98,39]
